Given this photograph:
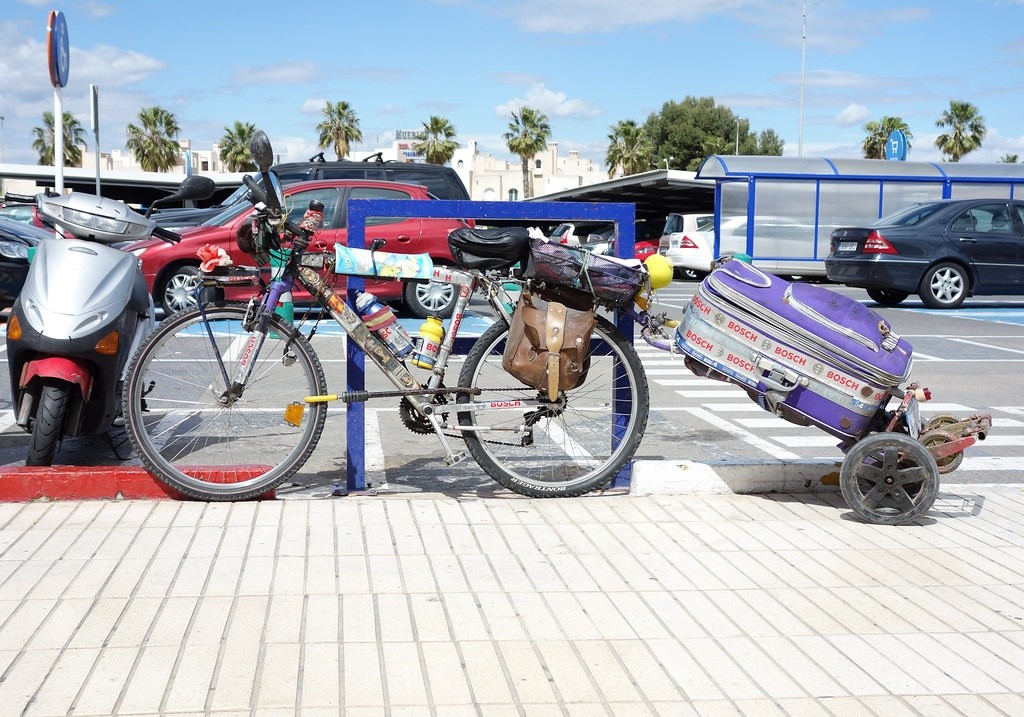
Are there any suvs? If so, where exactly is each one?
[139,152,470,236]
[120,179,477,321]
[656,212,737,279]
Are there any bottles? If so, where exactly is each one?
[354,290,414,356]
[411,310,444,369]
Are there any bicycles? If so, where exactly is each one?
[120,129,652,504]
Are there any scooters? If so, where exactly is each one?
[0,174,217,467]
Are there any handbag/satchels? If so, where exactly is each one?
[530,236,646,304]
[501,294,597,401]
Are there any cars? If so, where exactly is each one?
[665,216,827,277]
[547,221,662,262]
[0,193,83,316]
[824,198,1024,309]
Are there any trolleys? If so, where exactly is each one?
[683,358,995,529]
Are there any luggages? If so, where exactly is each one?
[640,255,916,440]
